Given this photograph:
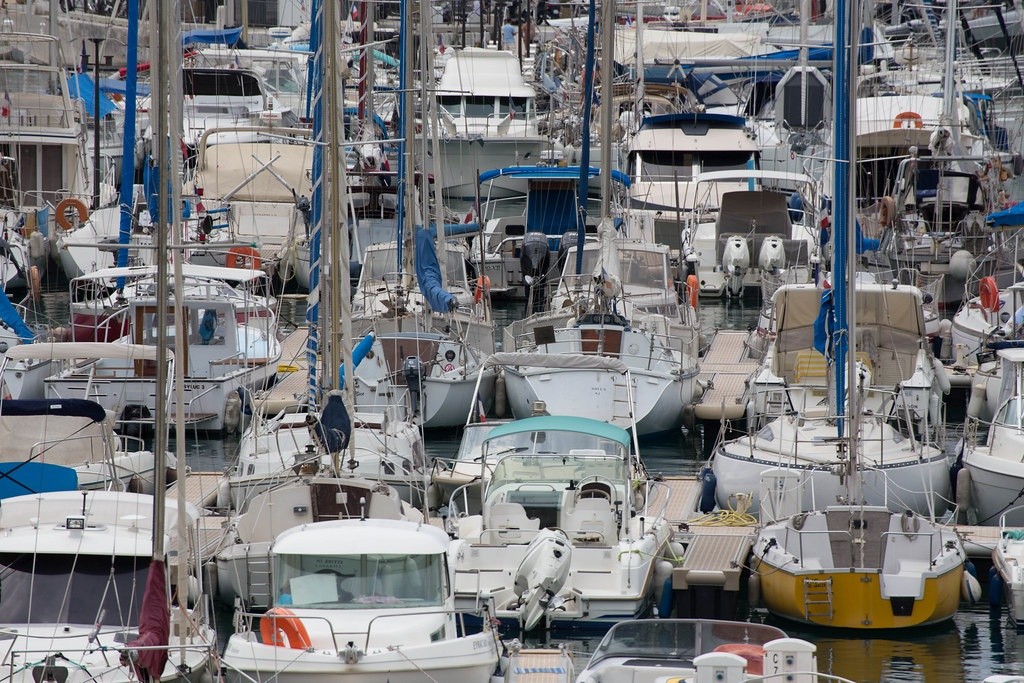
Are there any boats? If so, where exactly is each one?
[0,0,1024,682]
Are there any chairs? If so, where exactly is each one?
[757,235,786,272]
[723,235,750,274]
[568,489,619,543]
[491,503,541,543]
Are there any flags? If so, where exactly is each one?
[2,90,12,119]
[60,72,118,120]
[351,5,358,20]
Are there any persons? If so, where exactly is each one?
[501,14,535,55]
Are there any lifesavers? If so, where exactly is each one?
[258,606,312,652]
[879,195,895,226]
[978,276,999,311]
[687,274,699,308]
[225,245,260,270]
[475,275,492,302]
[55,200,88,228]
[892,112,923,128]
